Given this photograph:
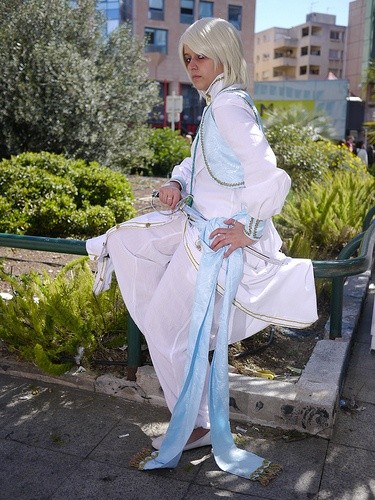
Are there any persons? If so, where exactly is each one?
[337,131,375,167]
[85,16,319,486]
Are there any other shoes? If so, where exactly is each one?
[151,429,211,450]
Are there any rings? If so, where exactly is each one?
[168,197,173,199]
[232,219,238,226]
